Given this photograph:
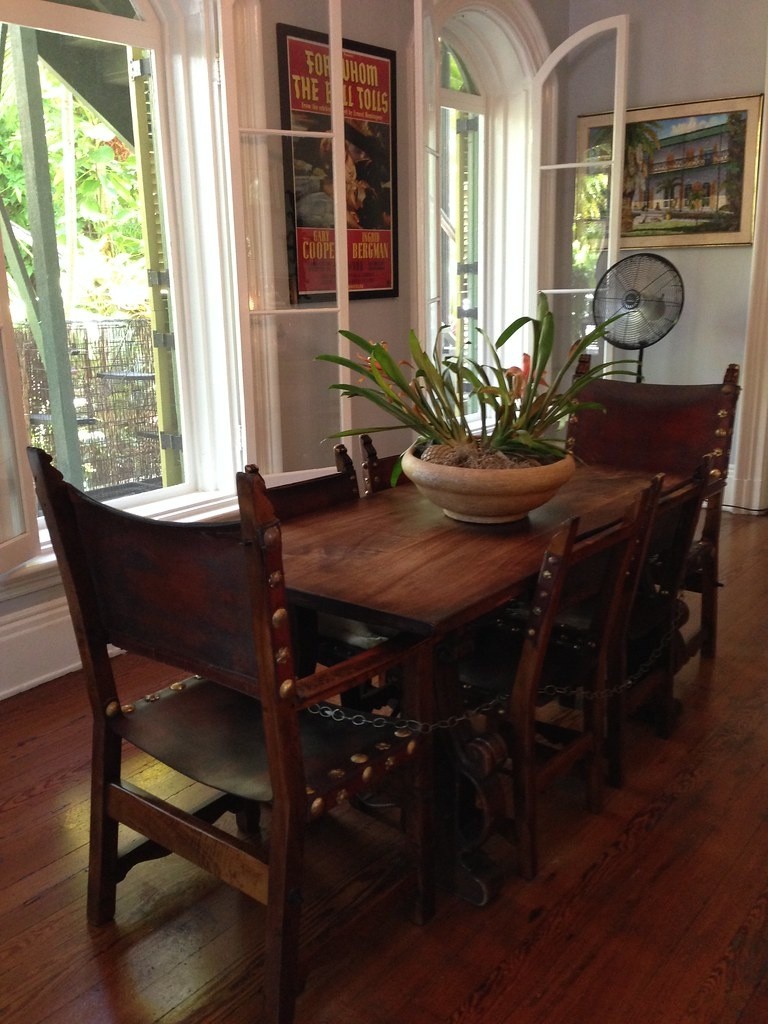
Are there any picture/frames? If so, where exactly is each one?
[571,93,765,254]
[276,22,401,308]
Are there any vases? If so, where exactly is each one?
[402,439,578,525]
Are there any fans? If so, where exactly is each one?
[592,251,685,384]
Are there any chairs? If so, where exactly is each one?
[23,355,743,1021]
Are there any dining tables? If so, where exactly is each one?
[276,471,727,923]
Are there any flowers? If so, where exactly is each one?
[308,292,649,490]
